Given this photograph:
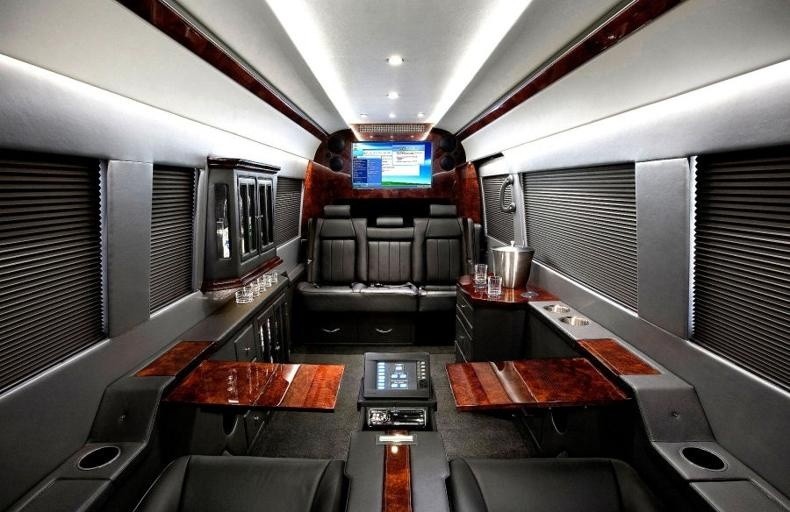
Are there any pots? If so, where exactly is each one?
[489,245,535,289]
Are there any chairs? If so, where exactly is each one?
[127,454,351,512]
[294,203,368,312]
[450,458,656,512]
[369,217,418,314]
[413,203,481,313]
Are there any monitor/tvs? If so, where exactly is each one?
[364,352,430,398]
[351,140,433,190]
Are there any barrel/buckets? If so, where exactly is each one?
[491,239,535,290]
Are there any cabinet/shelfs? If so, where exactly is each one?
[451,282,529,363]
[202,158,284,292]
[297,313,418,346]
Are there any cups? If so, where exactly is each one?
[475,263,488,284]
[235,270,278,303]
[488,276,503,296]
